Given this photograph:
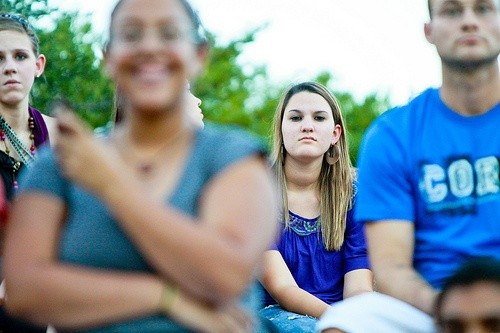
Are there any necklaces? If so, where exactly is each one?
[0,117,35,189]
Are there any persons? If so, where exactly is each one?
[255,81,373,333]
[433,259,500,333]
[0,0,282,333]
[316,0,500,333]
